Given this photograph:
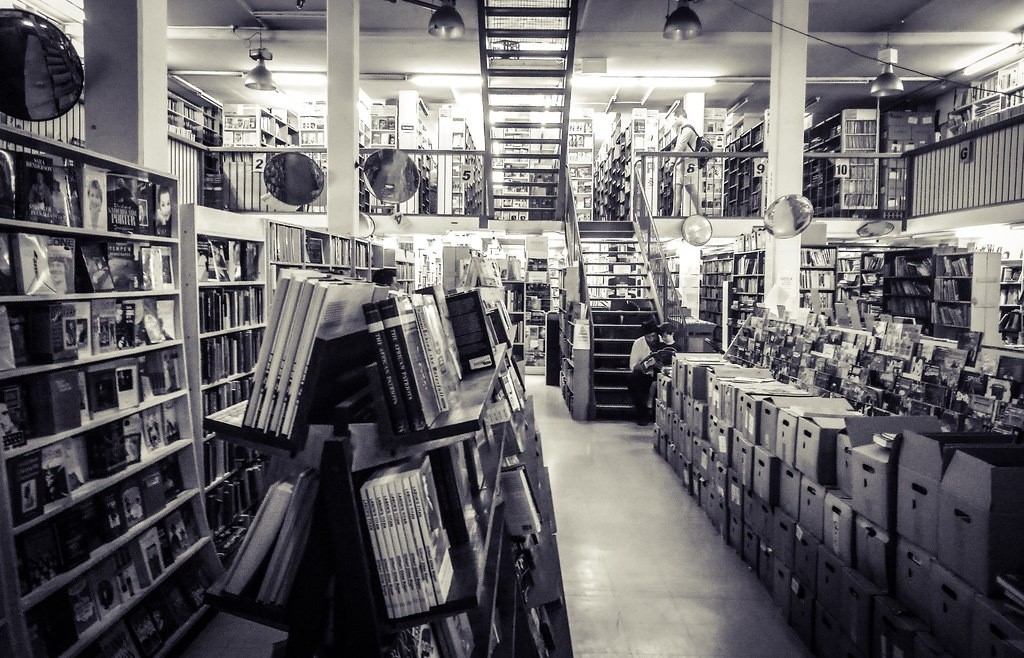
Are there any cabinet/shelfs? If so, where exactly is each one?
[837,245,1024,346]
[948,58,1024,137]
[723,112,879,217]
[0,0,733,658]
[723,304,1024,437]
[734,245,837,336]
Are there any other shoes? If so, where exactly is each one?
[637,418,649,425]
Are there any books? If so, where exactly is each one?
[192,223,587,658]
[165,95,878,219]
[947,63,1024,137]
[582,235,682,320]
[698,243,1023,458]
[1,149,205,658]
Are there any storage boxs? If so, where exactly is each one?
[731,225,828,251]
[880,109,937,210]
[651,353,1024,658]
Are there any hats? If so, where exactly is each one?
[640,318,658,334]
[657,322,677,334]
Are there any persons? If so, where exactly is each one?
[629,319,682,426]
[664,108,703,218]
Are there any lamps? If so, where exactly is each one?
[871,25,905,98]
[605,97,616,115]
[803,98,822,111]
[357,73,410,81]
[662,0,704,41]
[726,96,751,115]
[243,28,279,93]
[663,101,680,120]
[426,0,467,40]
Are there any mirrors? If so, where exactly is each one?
[763,194,815,240]
[680,213,713,246]
[859,220,895,238]
[0,8,85,122]
[263,152,325,206]
[359,210,375,238]
[362,148,420,203]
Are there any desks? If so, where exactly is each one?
[669,316,717,353]
